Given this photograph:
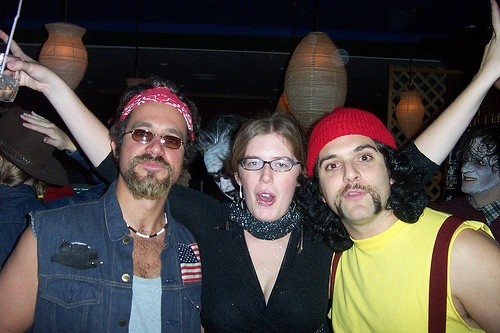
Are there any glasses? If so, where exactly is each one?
[121,129,185,150]
[237,157,303,172]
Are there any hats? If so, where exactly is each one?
[307,106,400,178]
[0,106,69,187]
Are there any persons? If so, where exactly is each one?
[305,106,500,333]
[194,115,251,203]
[0,106,109,333]
[0,0,500,332]
[421,120,499,244]
[1,78,206,333]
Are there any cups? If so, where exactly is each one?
[0,53,24,102]
